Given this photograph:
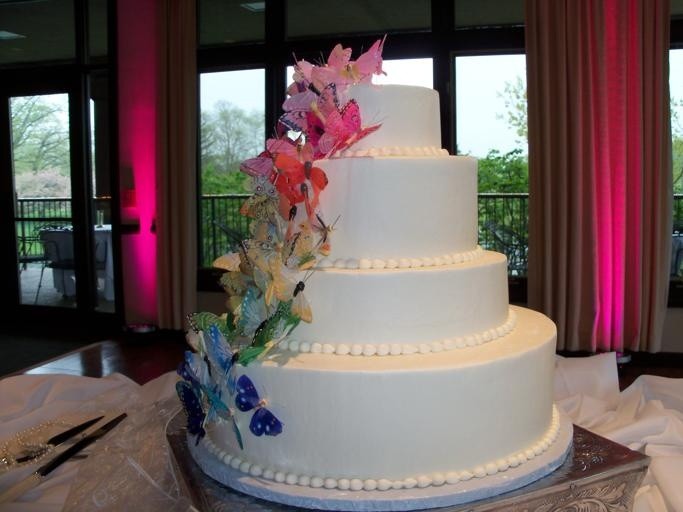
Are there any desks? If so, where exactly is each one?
[0,325,682,512]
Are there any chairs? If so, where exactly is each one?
[16,232,102,306]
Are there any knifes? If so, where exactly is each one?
[0,412,130,502]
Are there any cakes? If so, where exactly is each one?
[176,33,560,491]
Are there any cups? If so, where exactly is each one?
[97,210,104,227]
